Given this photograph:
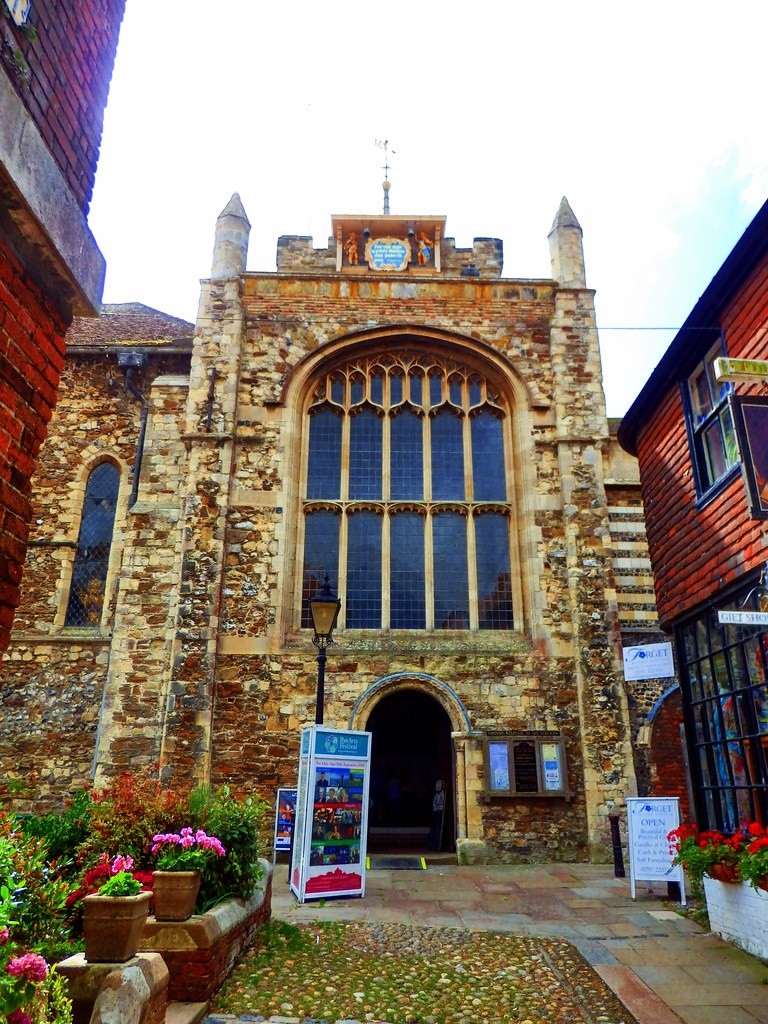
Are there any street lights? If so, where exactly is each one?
[305,572,343,725]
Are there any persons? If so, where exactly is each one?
[278,802,293,837]
[312,770,362,865]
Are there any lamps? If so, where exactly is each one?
[406,221,416,238]
[361,220,373,238]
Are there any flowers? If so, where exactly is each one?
[745,823,768,879]
[152,828,224,874]
[666,822,745,877]
[93,856,157,895]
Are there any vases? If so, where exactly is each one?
[711,858,745,884]
[754,873,768,892]
[152,867,206,920]
[84,892,154,962]
